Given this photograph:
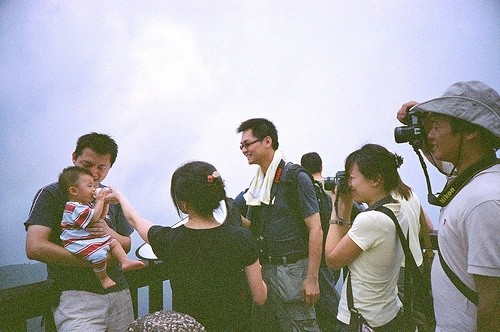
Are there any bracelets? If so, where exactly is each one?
[329,220,344,226]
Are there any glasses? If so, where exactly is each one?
[239,139,261,150]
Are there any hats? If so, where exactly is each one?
[409,80,500,152]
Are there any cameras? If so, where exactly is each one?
[324,171,349,193]
[394,113,425,149]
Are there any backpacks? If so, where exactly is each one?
[286,165,333,269]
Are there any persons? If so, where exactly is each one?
[301,152,345,332]
[57,166,144,289]
[387,151,433,332]
[105,161,268,332]
[396,81,499,332]
[324,144,413,332]
[23,131,136,332]
[236,118,324,332]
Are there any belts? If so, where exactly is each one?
[260,251,309,265]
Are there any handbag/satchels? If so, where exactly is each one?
[345,205,437,332]
